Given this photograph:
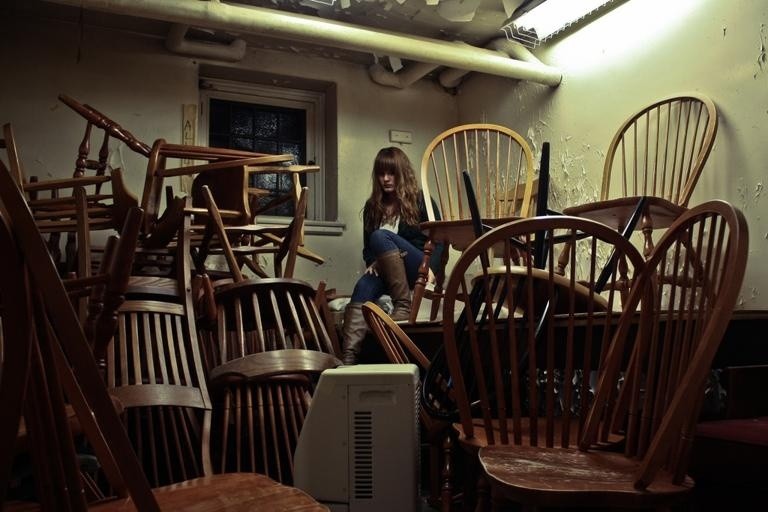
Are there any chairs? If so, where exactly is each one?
[550,93,719,312]
[1,93,347,511]
[360,199,659,512]
[408,122,536,326]
[477,198,752,511]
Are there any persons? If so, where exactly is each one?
[339,147,443,366]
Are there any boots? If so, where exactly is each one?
[339,300,371,365]
[377,247,413,321]
